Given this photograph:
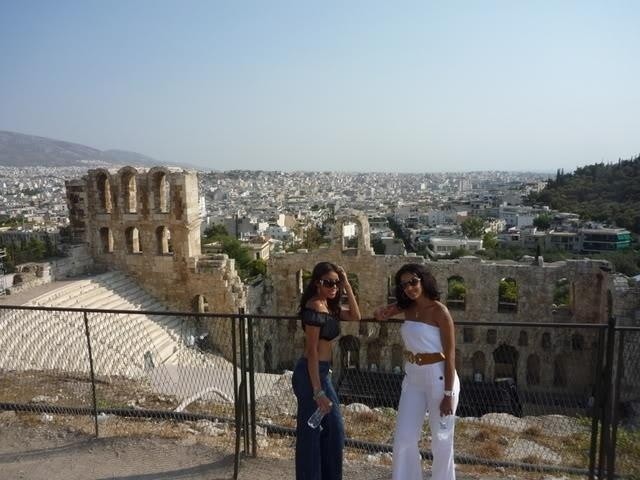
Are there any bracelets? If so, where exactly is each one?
[312,388,325,400]
[444,390,456,397]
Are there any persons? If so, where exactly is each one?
[290,261,362,480]
[372,262,462,480]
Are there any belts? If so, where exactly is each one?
[401,347,446,365]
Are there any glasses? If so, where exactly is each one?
[398,274,422,290]
[315,277,342,289]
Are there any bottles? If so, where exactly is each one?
[437,415,450,441]
[307,405,325,429]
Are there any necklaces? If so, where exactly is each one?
[414,307,421,318]
